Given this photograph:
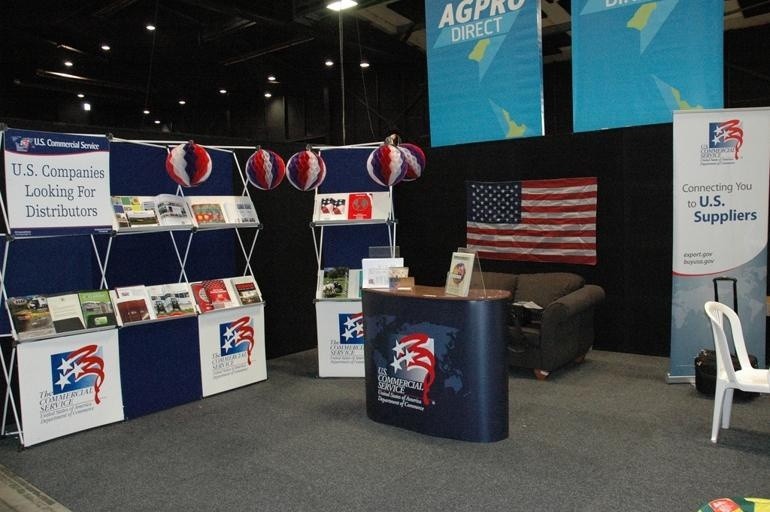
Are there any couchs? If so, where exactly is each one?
[470,271,606,381]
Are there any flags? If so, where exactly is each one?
[465,176,598,267]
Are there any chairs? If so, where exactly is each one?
[704,301,770,444]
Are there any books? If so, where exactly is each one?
[443,251,474,298]
[316,266,363,298]
[317,192,373,220]
[5,272,261,341]
[109,195,260,232]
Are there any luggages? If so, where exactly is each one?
[694,278,760,401]
